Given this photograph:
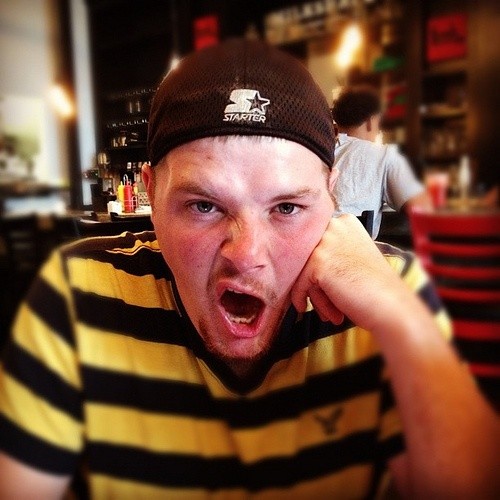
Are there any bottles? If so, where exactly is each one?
[115,160,149,214]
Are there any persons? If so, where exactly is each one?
[327,89,435,241]
[0,39,500,500]
[468,68,500,208]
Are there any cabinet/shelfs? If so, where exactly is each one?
[104,88,156,151]
[403,0,500,196]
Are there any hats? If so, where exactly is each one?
[145,42,336,167]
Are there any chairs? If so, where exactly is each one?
[405,204,500,379]
